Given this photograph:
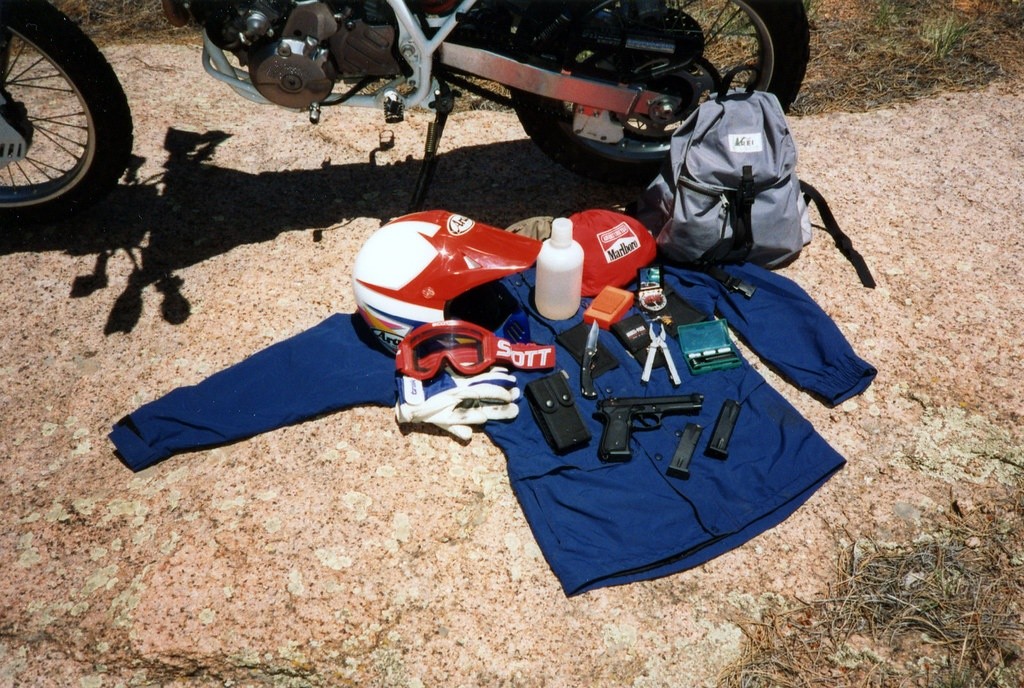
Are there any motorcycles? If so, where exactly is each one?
[1,1,811,228]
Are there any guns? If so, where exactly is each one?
[595,392,705,463]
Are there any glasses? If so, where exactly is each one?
[396,320,498,380]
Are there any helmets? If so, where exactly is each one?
[352,210,543,357]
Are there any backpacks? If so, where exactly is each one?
[642,64,812,271]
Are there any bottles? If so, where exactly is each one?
[534,217,584,320]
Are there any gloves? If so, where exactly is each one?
[395,366,520,440]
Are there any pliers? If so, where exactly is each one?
[642,322,682,386]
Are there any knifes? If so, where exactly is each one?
[580,319,600,399]
